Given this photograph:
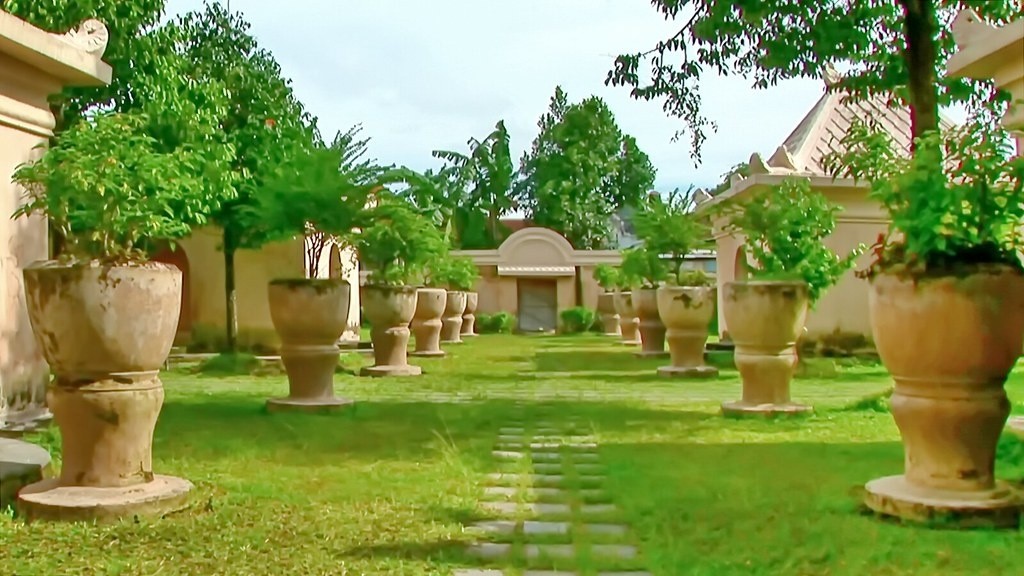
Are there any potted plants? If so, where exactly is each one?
[9,66,1024,487]
